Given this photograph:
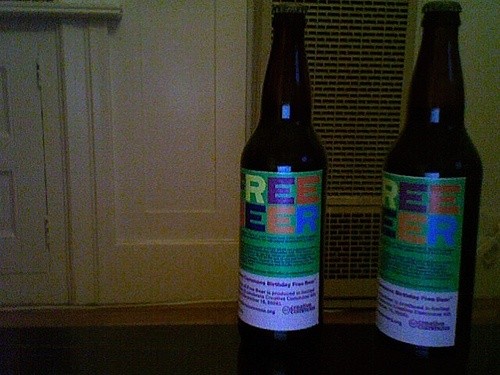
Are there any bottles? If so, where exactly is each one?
[238,4,328,339]
[375,1,483,359]
